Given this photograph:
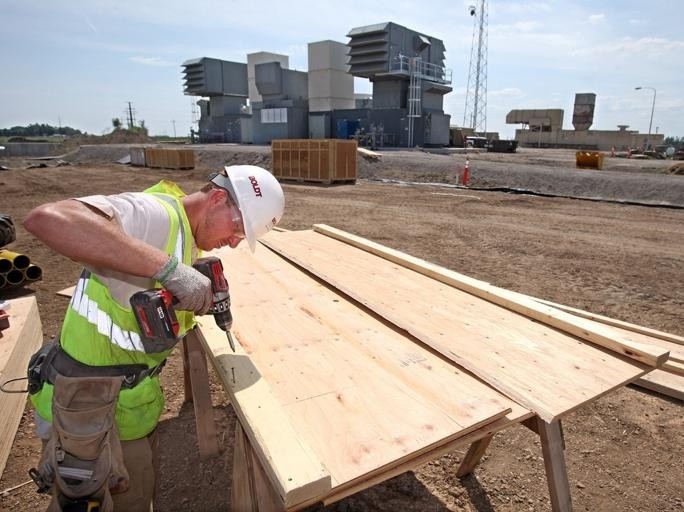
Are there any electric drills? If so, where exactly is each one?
[130,256,236,353]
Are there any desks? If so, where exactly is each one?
[179,221,670,512]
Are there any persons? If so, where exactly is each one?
[11,164,287,512]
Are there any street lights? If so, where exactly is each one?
[635,85,658,154]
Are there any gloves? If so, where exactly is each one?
[152,253,213,317]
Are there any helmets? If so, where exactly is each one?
[223,164,285,252]
